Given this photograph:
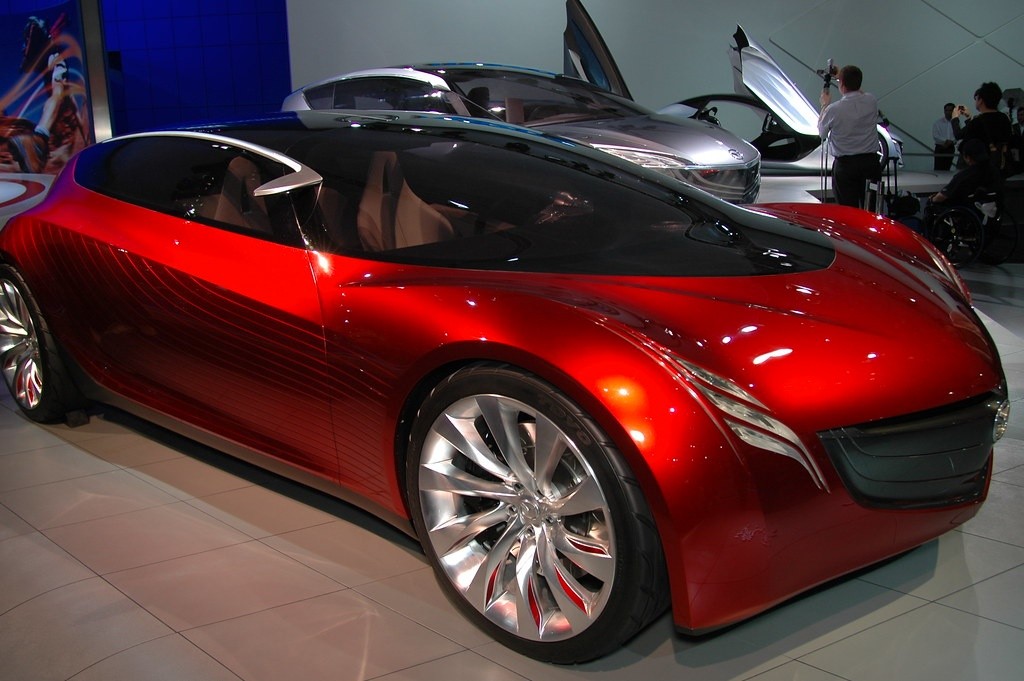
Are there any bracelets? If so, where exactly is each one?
[821,103,829,108]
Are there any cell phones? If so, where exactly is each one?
[958,106,965,113]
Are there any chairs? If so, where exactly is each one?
[356,149,454,254]
[213,153,272,237]
[466,86,490,118]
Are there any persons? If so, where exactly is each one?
[817,65,882,213]
[923,81,1024,243]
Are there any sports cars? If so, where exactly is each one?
[652,21,904,179]
[276,1,764,234]
[0,104,1013,665]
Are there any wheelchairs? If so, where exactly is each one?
[923,187,1019,271]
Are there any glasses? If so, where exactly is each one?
[836,78,841,82]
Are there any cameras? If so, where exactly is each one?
[817,58,837,82]
